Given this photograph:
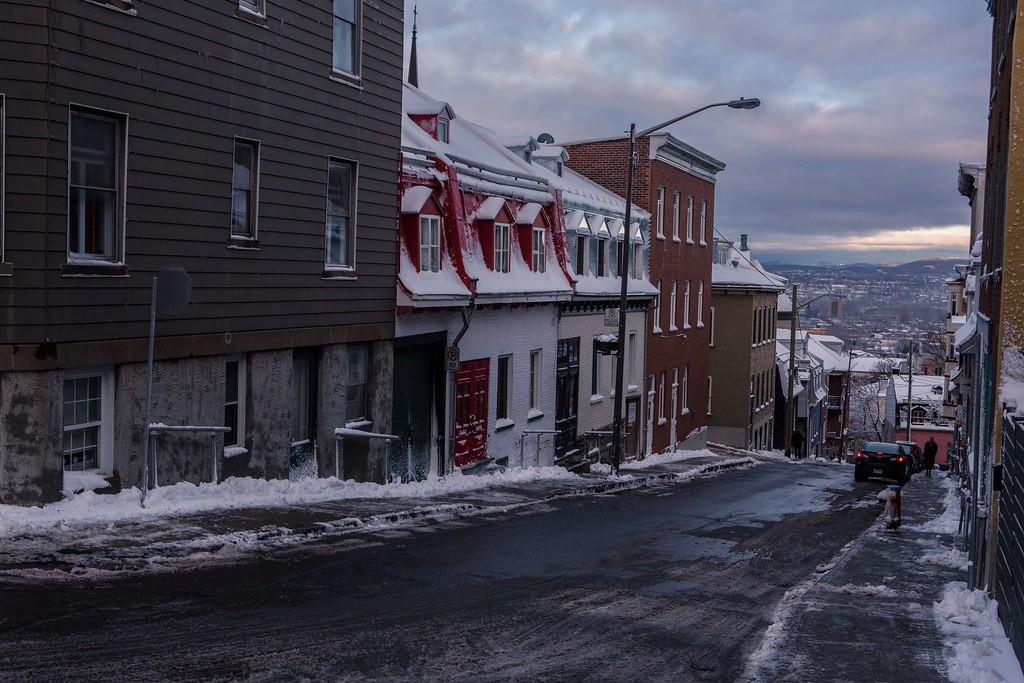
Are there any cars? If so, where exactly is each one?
[854,441,913,486]
[894,440,925,473]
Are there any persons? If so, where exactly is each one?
[923,437,938,476]
[791,430,805,459]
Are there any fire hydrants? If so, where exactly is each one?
[880,485,902,530]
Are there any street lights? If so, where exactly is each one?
[891,338,923,440]
[610,96,762,477]
[784,284,847,457]
